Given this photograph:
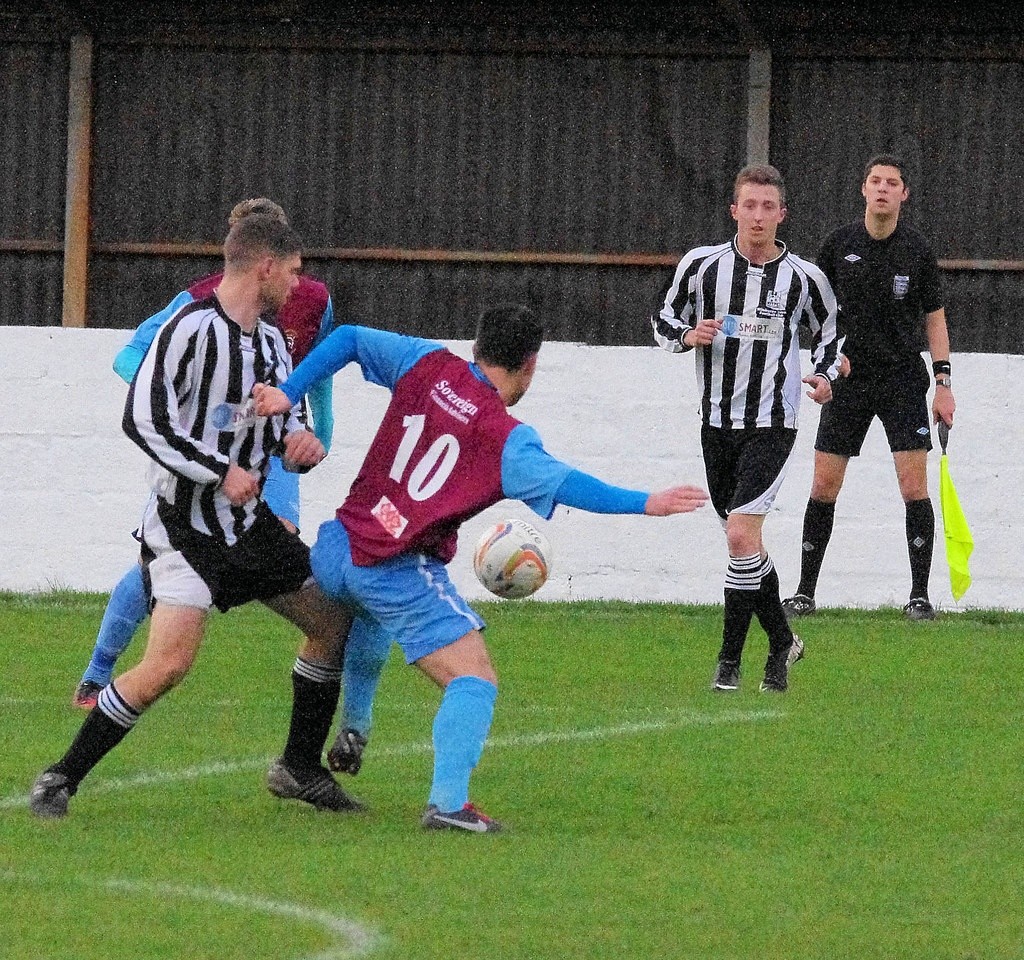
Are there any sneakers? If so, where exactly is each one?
[422,802,503,833]
[712,659,739,691]
[903,597,935,620]
[31,772,67,816]
[759,632,804,694]
[267,756,360,812]
[72,679,104,711]
[327,728,367,774]
[781,595,816,617]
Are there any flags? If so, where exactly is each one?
[937,452,973,601]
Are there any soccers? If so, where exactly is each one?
[473,518,553,598]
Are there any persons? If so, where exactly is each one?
[780,156,958,625]
[25,215,362,813]
[652,164,849,694]
[71,200,332,709]
[255,298,712,838]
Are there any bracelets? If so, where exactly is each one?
[931,360,952,376]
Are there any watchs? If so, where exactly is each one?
[934,377,952,389]
